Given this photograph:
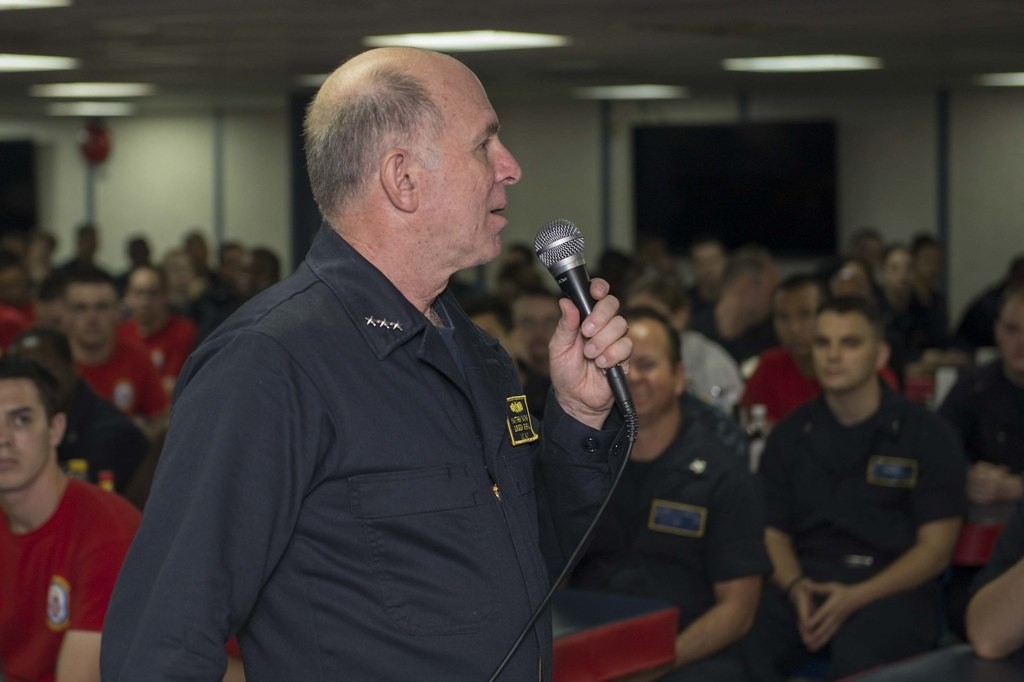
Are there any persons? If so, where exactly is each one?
[0,353,145,682]
[931,278,1024,500]
[98,46,641,682]
[741,270,898,428]
[1,222,284,500]
[465,224,1005,395]
[962,500,1024,681]
[750,294,970,682]
[534,304,776,682]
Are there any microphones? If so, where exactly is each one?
[534,219,638,442]
